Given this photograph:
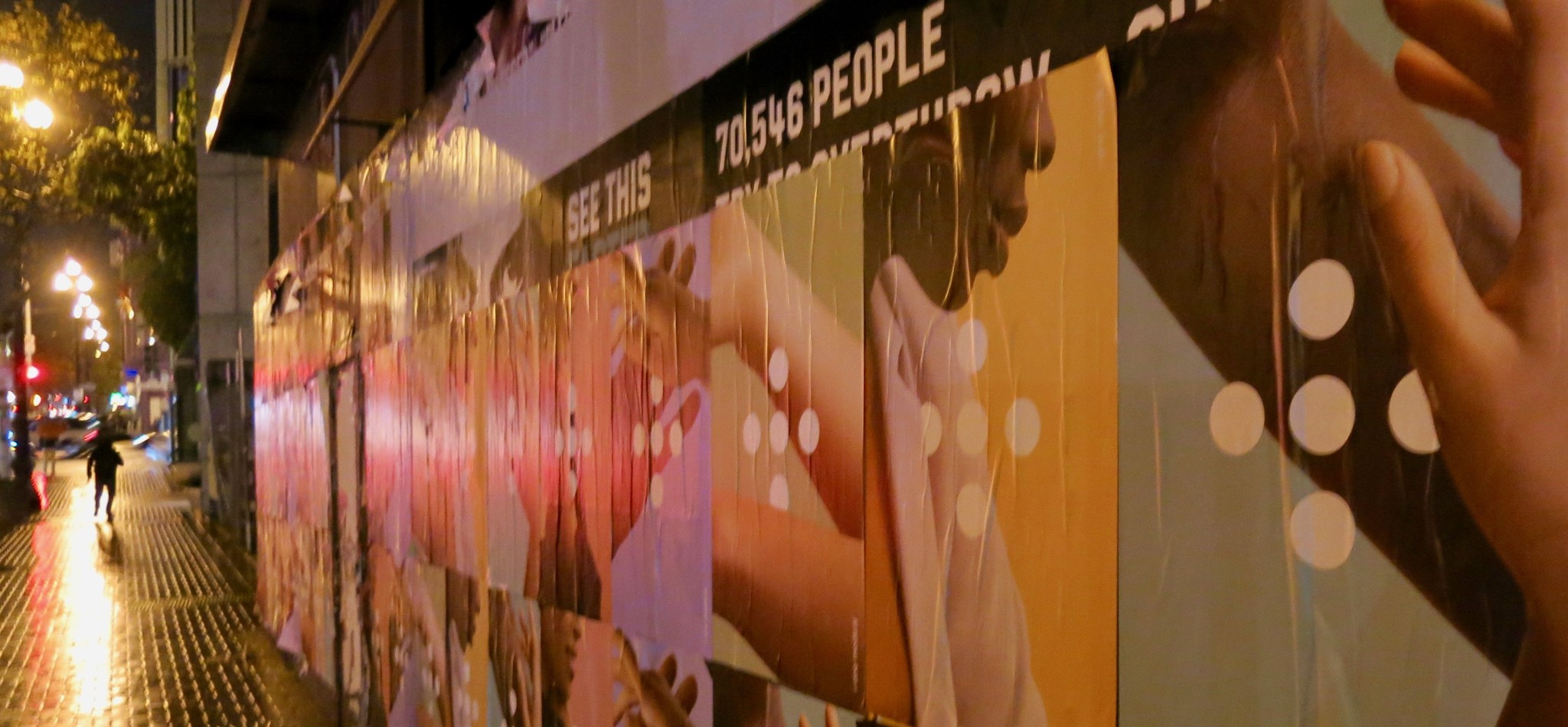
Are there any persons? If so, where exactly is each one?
[38,413,64,476]
[617,210,709,628]
[1118,1,1568,725]
[87,433,124,517]
[541,605,701,727]
[367,554,540,726]
[414,220,615,621]
[861,76,1056,727]
[710,204,863,705]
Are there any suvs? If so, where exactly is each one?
[29,413,101,456]
